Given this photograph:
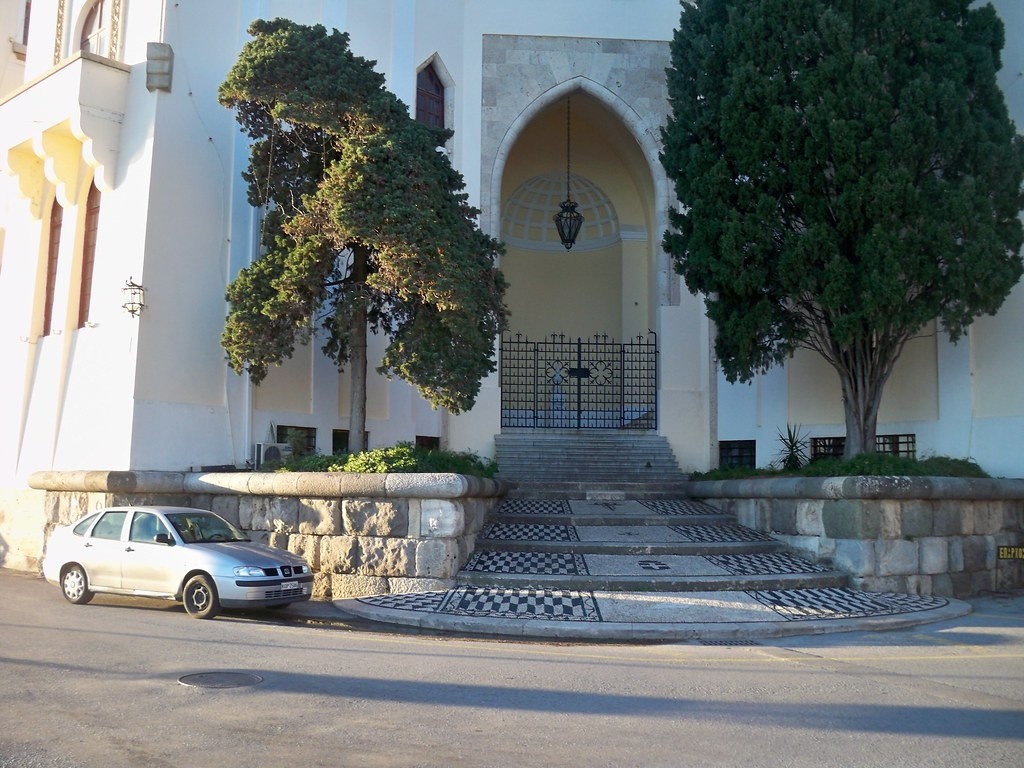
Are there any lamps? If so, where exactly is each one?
[552,94,585,251]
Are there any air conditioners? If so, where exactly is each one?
[254,442,292,470]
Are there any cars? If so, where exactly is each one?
[41,504,314,620]
